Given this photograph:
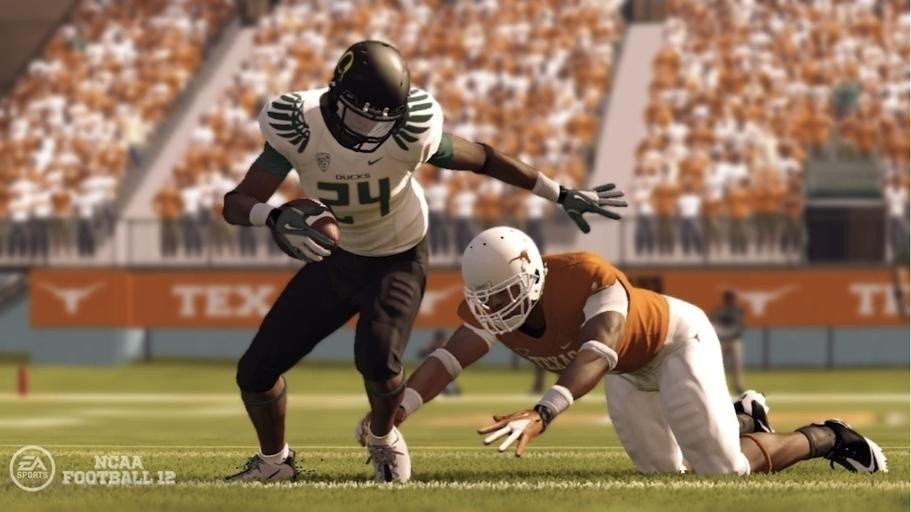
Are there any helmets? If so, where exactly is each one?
[324,40,411,154]
[461,226,548,335]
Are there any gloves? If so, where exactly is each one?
[557,183,630,233]
[265,203,336,266]
[478,404,553,457]
[356,405,407,448]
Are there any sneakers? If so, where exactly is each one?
[364,422,411,484]
[733,389,774,433]
[811,417,889,475]
[223,449,297,483]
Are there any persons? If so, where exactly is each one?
[356,224,892,478]
[1,0,909,268]
[222,42,629,484]
